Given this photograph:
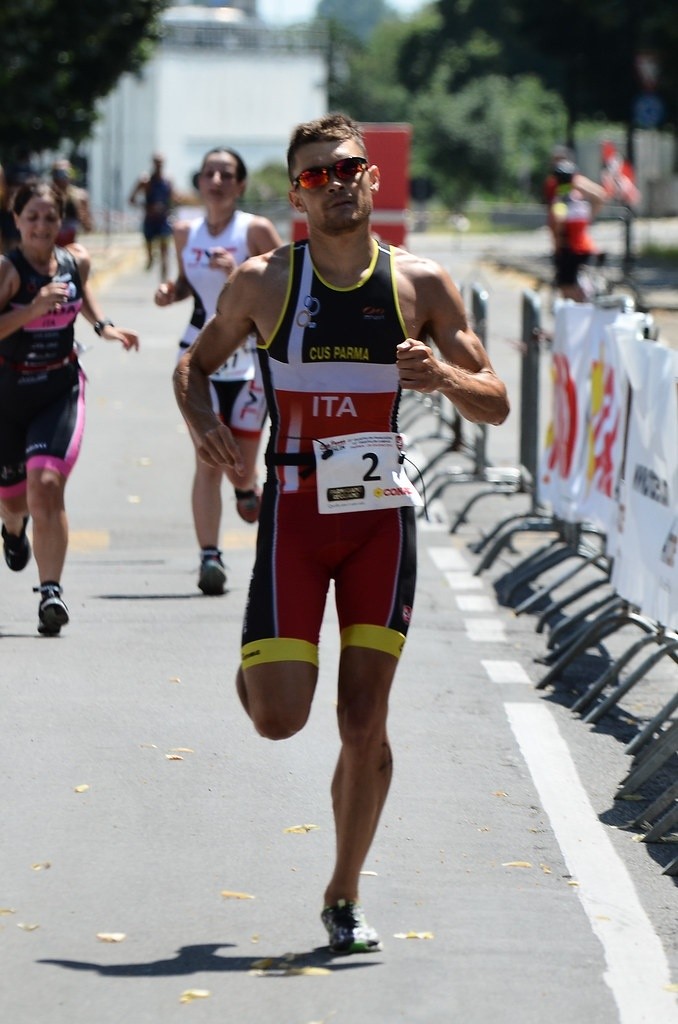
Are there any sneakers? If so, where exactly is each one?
[33,586,70,635]
[234,485,261,522]
[2,516,30,571]
[321,901,383,954]
[199,553,226,595]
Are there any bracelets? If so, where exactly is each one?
[94,320,113,336]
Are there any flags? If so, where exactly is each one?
[602,144,641,206]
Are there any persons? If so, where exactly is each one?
[129,159,175,283]
[173,113,511,954]
[155,147,282,595]
[1,179,138,635]
[547,140,606,303]
[51,160,92,248]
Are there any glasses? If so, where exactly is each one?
[292,157,371,191]
